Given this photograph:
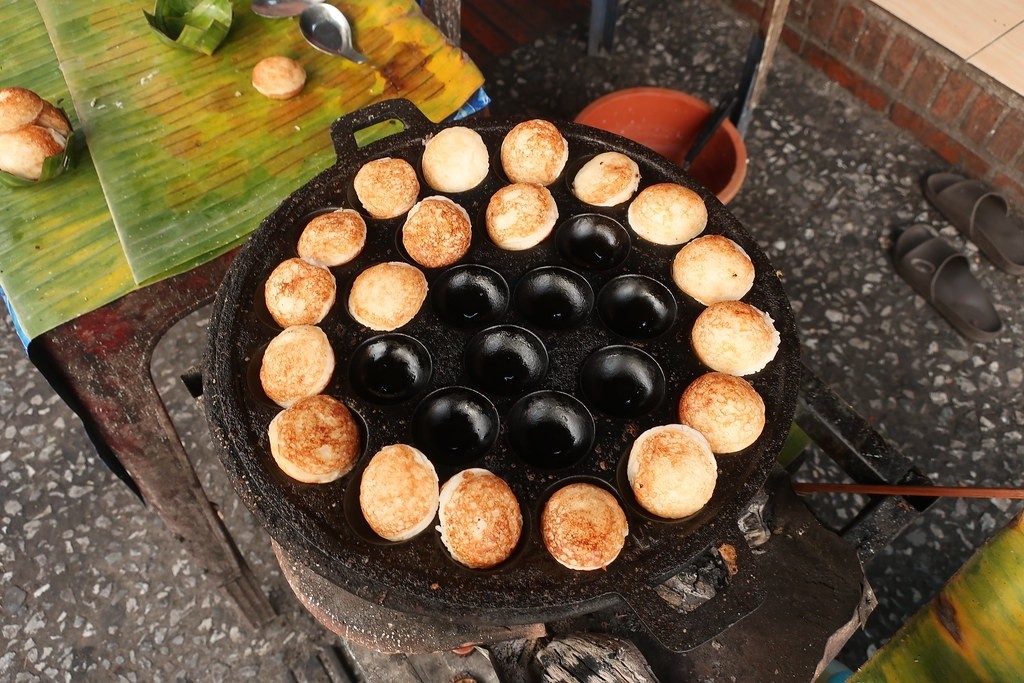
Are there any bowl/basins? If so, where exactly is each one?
[572,87,746,206]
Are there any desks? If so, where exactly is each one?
[0,0,490,626]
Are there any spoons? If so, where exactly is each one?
[297,2,368,65]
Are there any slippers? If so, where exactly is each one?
[926,173,1024,276]
[893,223,1005,342]
